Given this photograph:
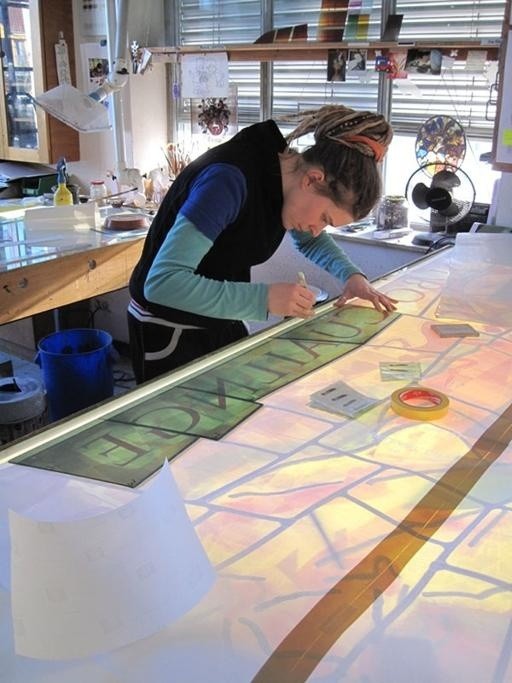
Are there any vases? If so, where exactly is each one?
[207,120,224,135]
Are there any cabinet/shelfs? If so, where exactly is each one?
[0,0,81,165]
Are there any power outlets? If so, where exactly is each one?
[95,300,107,309]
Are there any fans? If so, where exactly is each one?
[405,163,475,247]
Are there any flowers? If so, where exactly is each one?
[197,97,230,136]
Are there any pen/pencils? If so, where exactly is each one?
[298,272,309,291]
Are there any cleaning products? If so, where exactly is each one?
[54,157,74,206]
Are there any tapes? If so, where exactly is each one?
[391,387,450,421]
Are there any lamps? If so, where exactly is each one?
[30,0,128,135]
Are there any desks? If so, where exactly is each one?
[0,244,512,683]
[0,198,148,326]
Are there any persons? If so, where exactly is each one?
[91,62,104,78]
[123,102,401,385]
[331,51,345,80]
[406,51,431,68]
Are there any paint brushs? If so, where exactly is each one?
[88,187,137,204]
[161,143,191,179]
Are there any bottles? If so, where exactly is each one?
[89,179,108,206]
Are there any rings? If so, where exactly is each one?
[340,296,347,302]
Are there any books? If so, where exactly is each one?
[305,380,383,420]
[429,323,480,339]
[376,358,422,380]
